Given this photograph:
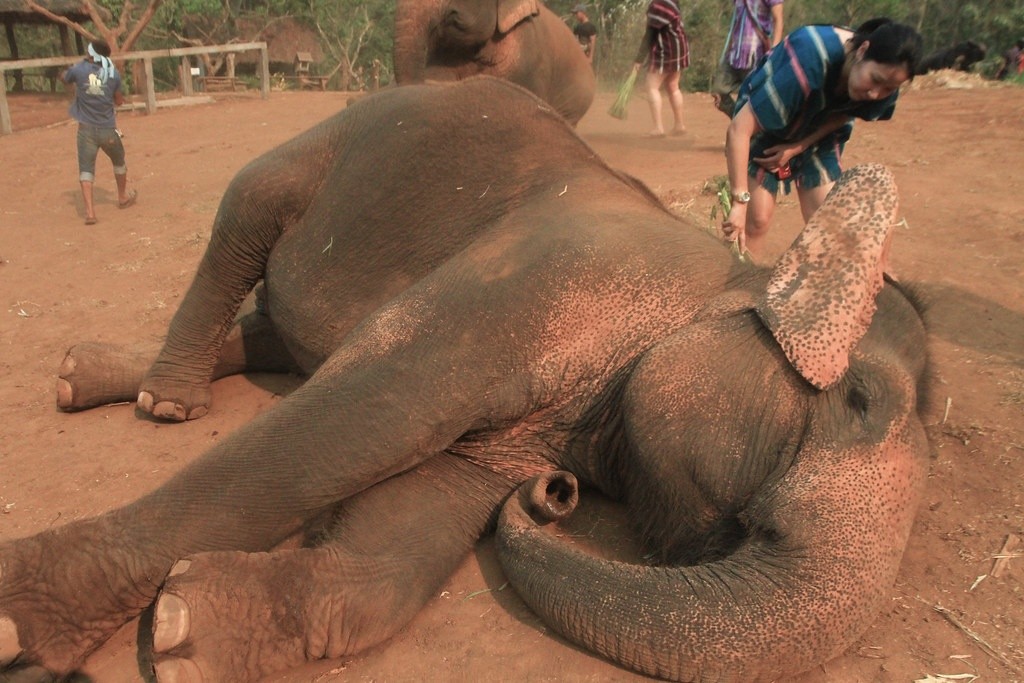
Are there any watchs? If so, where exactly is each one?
[731,191,750,203]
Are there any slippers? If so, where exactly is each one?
[85,215,97,225]
[119,189,136,208]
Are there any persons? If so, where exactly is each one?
[571,3,596,63]
[635,0,689,138]
[60,40,137,224]
[711,0,784,129]
[726,17,924,268]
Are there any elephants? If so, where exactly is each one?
[393,1,595,127]
[0,70,940,683]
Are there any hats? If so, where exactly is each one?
[570,4,585,13]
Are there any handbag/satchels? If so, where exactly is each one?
[763,36,771,55]
